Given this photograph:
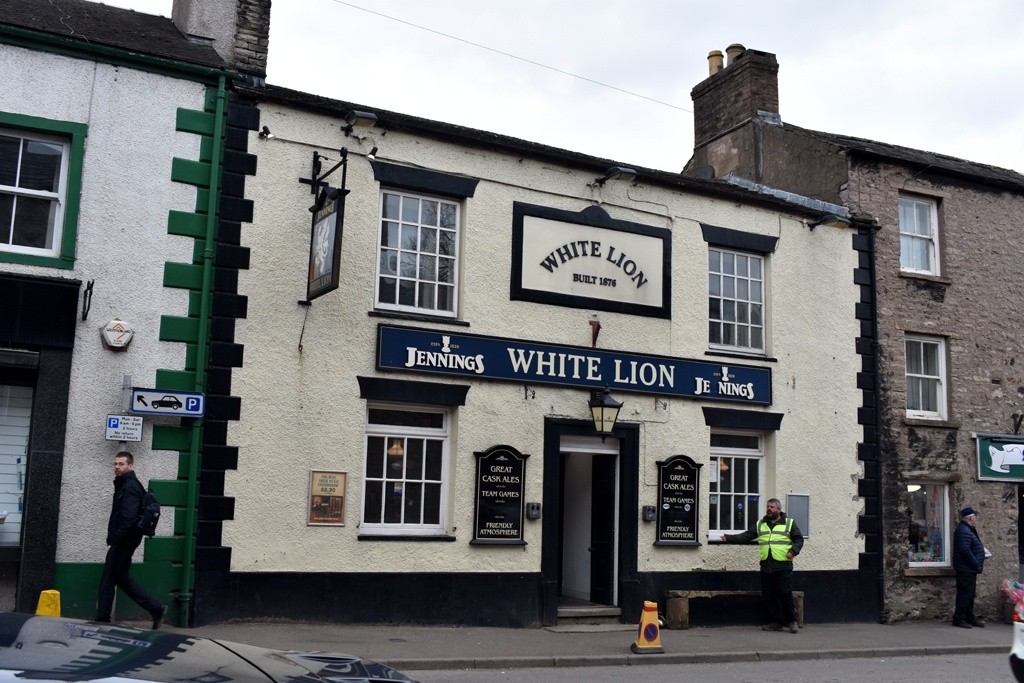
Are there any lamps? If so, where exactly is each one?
[366,147,378,163]
[341,110,377,136]
[588,384,624,444]
[595,167,637,188]
[807,215,850,231]
[388,439,403,456]
[259,125,274,142]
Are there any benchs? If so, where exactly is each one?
[665,590,803,629]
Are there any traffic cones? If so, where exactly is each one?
[630,599,667,655]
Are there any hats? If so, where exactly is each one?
[961,507,974,515]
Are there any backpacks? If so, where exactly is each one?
[125,478,160,535]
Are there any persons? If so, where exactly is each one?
[952,507,986,628]
[720,498,804,633]
[92,452,169,630]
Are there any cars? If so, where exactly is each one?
[1,610,419,683]
[1009,595,1024,683]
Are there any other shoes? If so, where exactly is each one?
[762,622,783,631]
[789,621,798,633]
[953,620,973,628]
[968,617,986,627]
[152,605,169,631]
